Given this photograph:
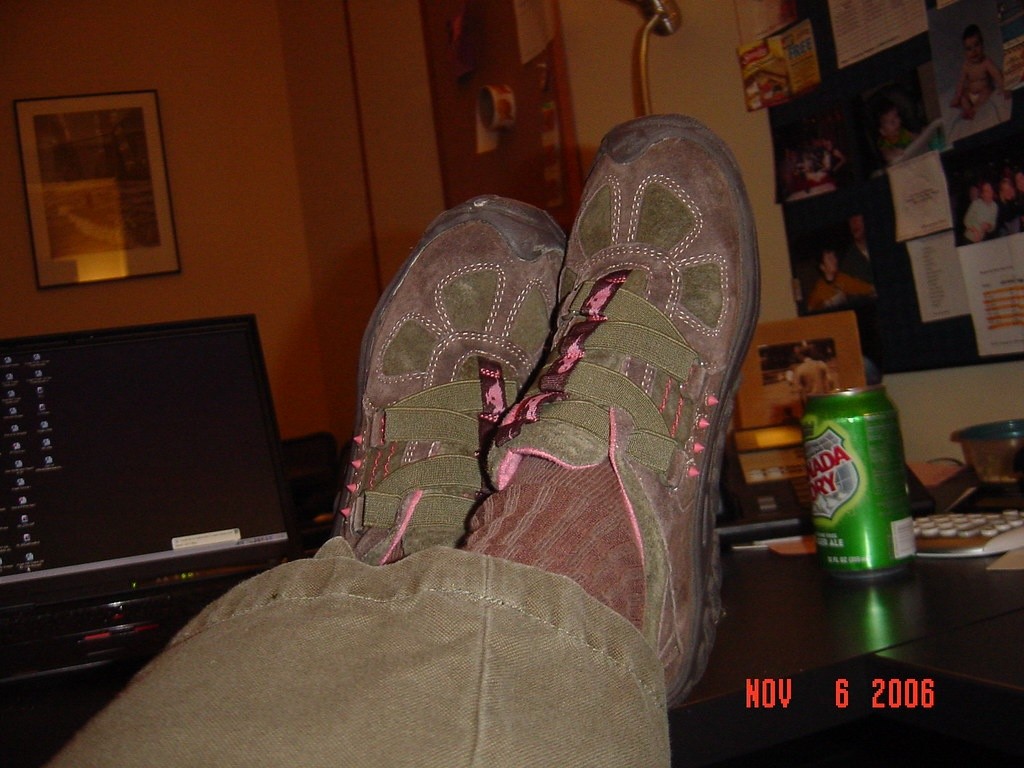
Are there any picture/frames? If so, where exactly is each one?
[14,90,180,291]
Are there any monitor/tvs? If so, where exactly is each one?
[0,314,306,617]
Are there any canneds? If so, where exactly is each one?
[798,383,916,579]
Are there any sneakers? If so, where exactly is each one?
[320,193,569,565]
[484,114,760,710]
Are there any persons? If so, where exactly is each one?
[791,345,837,413]
[799,210,894,308]
[49,113,763,768]
[955,167,1024,246]
[946,24,1011,130]
[776,135,844,199]
[873,103,943,169]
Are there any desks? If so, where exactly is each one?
[670,556,1024,710]
[875,607,1024,705]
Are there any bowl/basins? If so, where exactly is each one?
[950,420,1024,496]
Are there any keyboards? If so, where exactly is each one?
[0,593,198,655]
